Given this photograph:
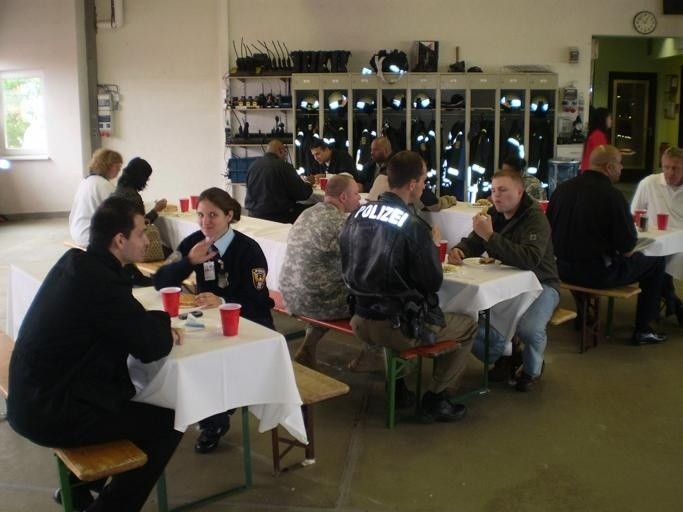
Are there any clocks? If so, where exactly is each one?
[633,10,656,35]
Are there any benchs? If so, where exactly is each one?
[266,289,457,430]
[63,241,206,292]
[481,293,578,383]
[544,269,642,355]
[258,360,351,475]
[0,332,148,510]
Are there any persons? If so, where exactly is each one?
[339,151,478,425]
[113,157,173,257]
[6,196,185,511]
[154,187,275,456]
[502,155,547,203]
[580,107,613,175]
[67,147,122,249]
[309,137,356,182]
[447,170,562,391]
[244,140,316,228]
[629,147,683,230]
[357,136,391,194]
[276,173,362,369]
[545,144,667,348]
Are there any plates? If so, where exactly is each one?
[170,320,205,330]
[462,258,501,266]
[441,263,460,274]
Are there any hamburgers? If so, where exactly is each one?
[179,294,197,308]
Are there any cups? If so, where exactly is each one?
[657,214,668,229]
[538,200,548,211]
[634,209,647,225]
[160,287,181,315]
[440,240,448,262]
[192,196,199,208]
[219,303,241,336]
[321,177,328,190]
[179,198,188,211]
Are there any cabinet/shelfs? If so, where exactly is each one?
[226,75,295,211]
[295,69,559,204]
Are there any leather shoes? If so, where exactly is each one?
[630,329,669,346]
[195,424,229,454]
[486,349,523,381]
[515,361,546,393]
[384,379,415,408]
[419,391,464,423]
